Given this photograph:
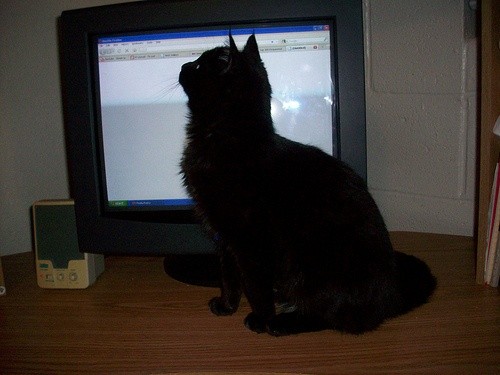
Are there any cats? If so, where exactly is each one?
[179,26,438,338]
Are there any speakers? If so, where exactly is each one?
[32,199,105,288]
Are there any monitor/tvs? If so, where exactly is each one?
[60,0,367,287]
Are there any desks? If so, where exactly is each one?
[0,231,500,375]
